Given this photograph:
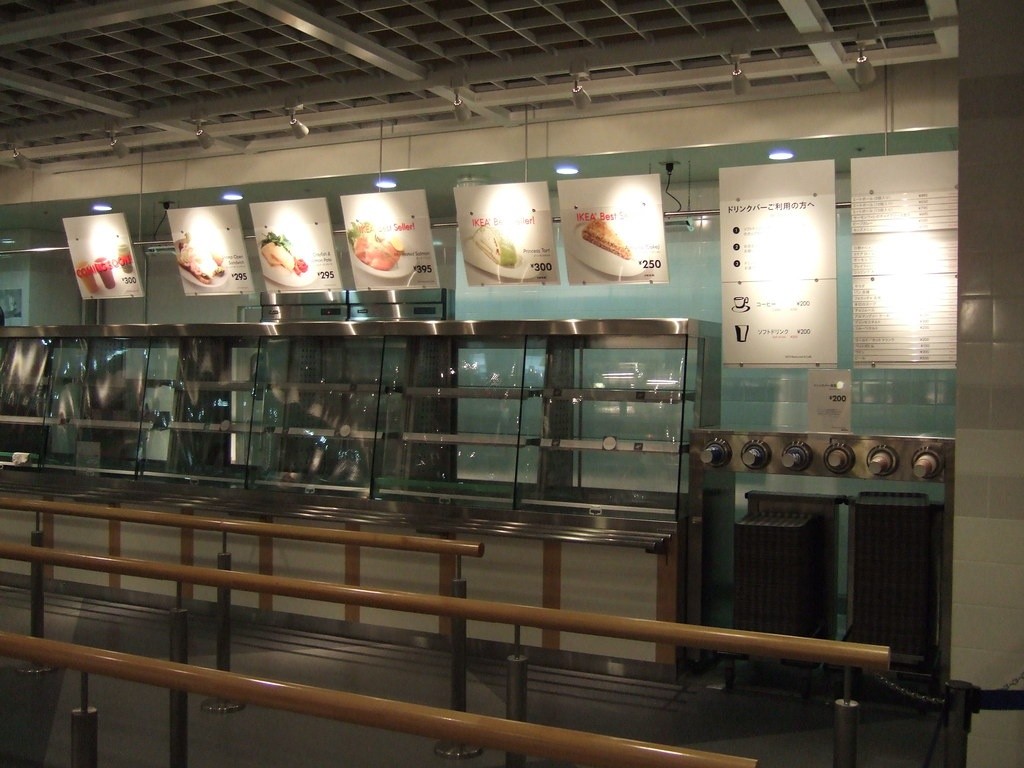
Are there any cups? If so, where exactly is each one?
[94,257,115,289]
[118,244,133,273]
[76,260,99,294]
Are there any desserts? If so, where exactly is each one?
[472,224,517,266]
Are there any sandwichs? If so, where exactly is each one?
[581,220,633,260]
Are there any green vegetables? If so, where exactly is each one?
[259,231,291,254]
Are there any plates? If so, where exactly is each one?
[462,225,541,279]
[257,226,318,287]
[346,219,417,278]
[174,237,229,288]
[564,219,650,277]
